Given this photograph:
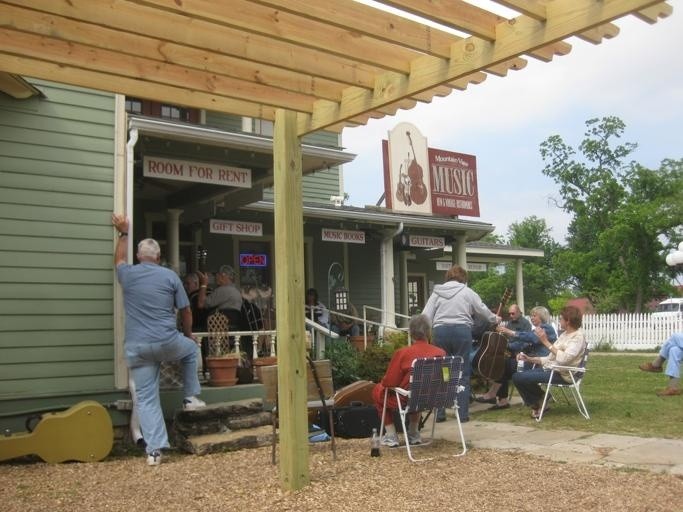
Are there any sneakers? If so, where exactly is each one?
[380,434,399,447]
[404,432,422,444]
[146,451,161,465]
[182,399,206,411]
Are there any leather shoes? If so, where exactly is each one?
[487,403,509,410]
[639,363,662,372]
[656,388,680,395]
[475,398,496,404]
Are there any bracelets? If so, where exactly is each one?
[548,345,553,350]
[511,331,516,336]
[200,283,208,287]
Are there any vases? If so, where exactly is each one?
[347,333,373,353]
[252,357,275,382]
[204,356,240,386]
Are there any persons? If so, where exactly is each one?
[370,314,447,448]
[421,263,503,422]
[636,333,683,397]
[469,305,558,410]
[192,263,243,313]
[502,302,531,357]
[330,299,359,337]
[304,288,328,329]
[108,211,206,468]
[510,304,588,419]
[180,269,199,294]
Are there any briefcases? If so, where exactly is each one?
[336,400,377,438]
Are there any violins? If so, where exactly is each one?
[396,163,412,206]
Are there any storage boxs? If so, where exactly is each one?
[317,400,378,439]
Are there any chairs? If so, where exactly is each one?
[376,356,470,465]
[509,374,570,410]
[520,350,590,421]
[259,357,340,464]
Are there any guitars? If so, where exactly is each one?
[472,287,513,379]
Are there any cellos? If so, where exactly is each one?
[406,131,427,204]
[188,244,218,379]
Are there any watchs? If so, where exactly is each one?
[118,231,128,237]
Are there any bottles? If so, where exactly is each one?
[515,350,524,373]
[368,426,380,456]
